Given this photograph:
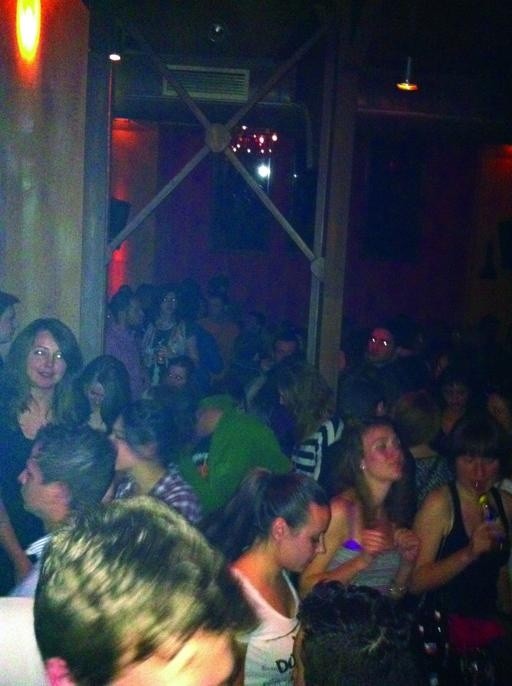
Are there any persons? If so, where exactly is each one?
[0,273,512,685]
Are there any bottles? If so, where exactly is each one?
[480,495,508,553]
